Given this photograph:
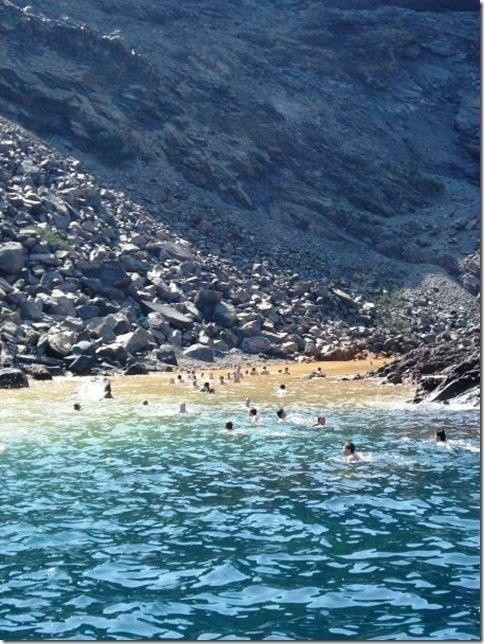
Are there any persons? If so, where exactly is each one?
[140,365,328,432]
[73,402,83,411]
[340,441,361,462]
[423,429,451,451]
